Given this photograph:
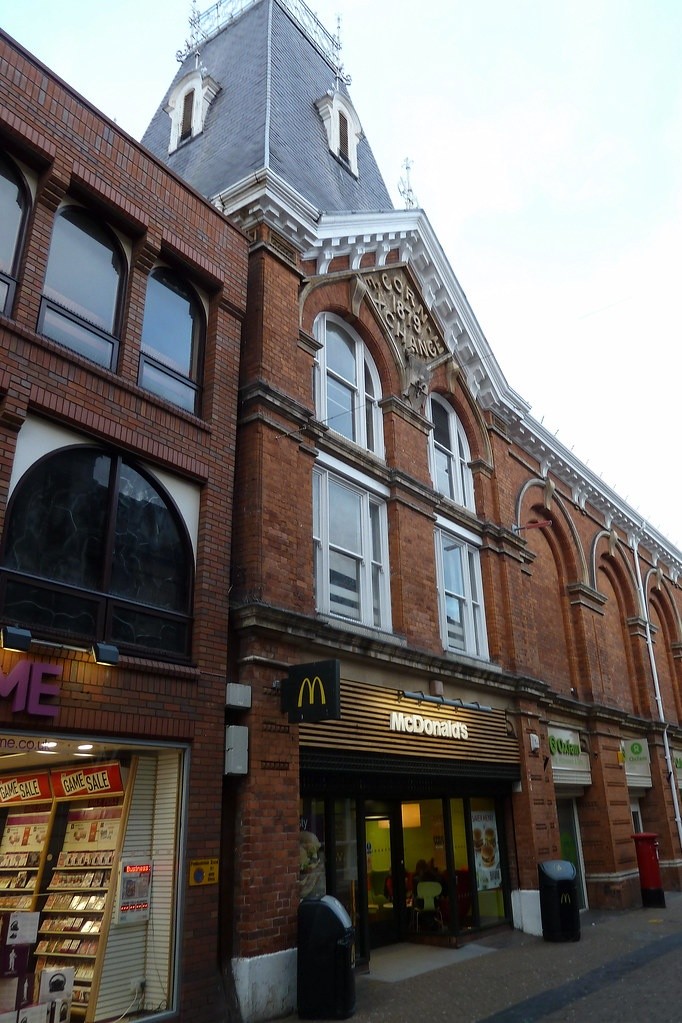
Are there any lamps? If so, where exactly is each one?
[1,625,32,653]
[512,520,552,534]
[401,801,421,828]
[86,641,120,666]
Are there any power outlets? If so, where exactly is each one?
[131,976,144,994]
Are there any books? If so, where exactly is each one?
[0,851,115,1002]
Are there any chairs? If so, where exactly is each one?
[411,881,445,934]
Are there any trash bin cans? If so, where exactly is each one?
[297,892,356,1021]
[537,860,580,943]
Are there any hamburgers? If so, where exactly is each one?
[472,828,496,866]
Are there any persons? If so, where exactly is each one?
[412,858,447,930]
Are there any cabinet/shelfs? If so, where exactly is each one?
[0,851,115,1023]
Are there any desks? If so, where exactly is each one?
[370,903,393,925]
